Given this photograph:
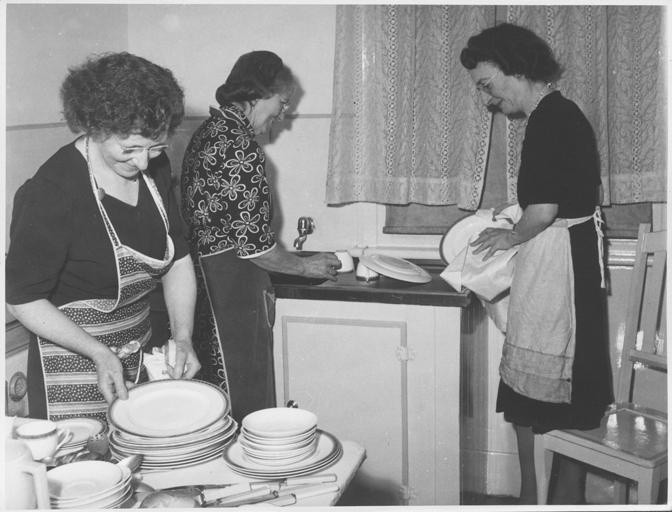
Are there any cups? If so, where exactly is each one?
[3,441,44,510]
[16,419,71,461]
[332,250,354,274]
[38,418,70,467]
[355,260,379,282]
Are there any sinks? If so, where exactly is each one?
[276,251,335,288]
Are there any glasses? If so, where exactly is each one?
[277,92,290,113]
[477,70,498,94]
[114,135,168,155]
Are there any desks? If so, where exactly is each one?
[6,414,367,506]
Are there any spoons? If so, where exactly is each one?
[115,452,149,469]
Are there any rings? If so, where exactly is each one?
[485,231,490,237]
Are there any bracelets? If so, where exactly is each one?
[510,224,520,245]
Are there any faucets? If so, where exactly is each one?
[293,217,313,250]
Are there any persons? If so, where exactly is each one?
[459,22,622,505]
[6,51,200,421]
[176,49,343,423]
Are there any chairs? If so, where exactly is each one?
[532,220,667,504]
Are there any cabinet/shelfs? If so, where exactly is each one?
[270,297,489,506]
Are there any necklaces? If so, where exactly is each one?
[522,81,553,127]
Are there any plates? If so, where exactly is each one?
[47,458,134,508]
[108,378,238,470]
[358,252,434,285]
[223,407,343,477]
[53,416,108,448]
[439,213,519,266]
